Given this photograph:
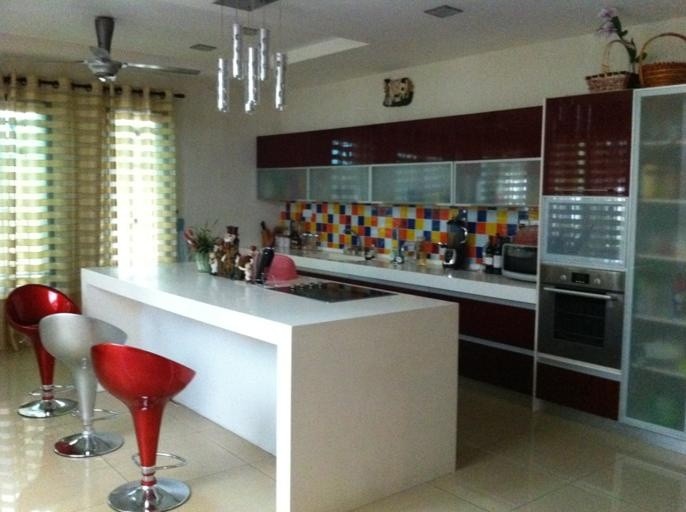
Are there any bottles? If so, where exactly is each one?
[247,245,260,276]
[226,225,239,249]
[259,220,272,247]
[484,234,502,275]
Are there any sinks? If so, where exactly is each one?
[327,253,366,262]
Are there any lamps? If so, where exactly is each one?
[209,0,291,115]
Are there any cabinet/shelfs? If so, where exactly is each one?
[541,89,629,197]
[454,106,542,210]
[617,83,685,441]
[431,288,536,397]
[309,127,370,204]
[371,116,454,209]
[535,356,621,423]
[257,135,308,200]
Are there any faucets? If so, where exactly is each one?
[342,228,362,249]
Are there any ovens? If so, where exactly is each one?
[534,261,626,374]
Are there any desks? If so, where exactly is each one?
[75,261,461,511]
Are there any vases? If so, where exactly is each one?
[194,251,213,274]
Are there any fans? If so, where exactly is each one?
[31,14,203,83]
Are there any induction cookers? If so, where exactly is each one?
[266,282,397,303]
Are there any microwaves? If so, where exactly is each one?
[501,242,537,282]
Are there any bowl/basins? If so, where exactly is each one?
[266,254,297,281]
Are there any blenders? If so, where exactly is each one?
[443,220,471,269]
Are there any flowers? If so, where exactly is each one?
[593,4,648,76]
[181,218,219,261]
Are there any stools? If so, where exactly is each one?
[86,343,200,510]
[3,282,81,419]
[36,308,130,458]
